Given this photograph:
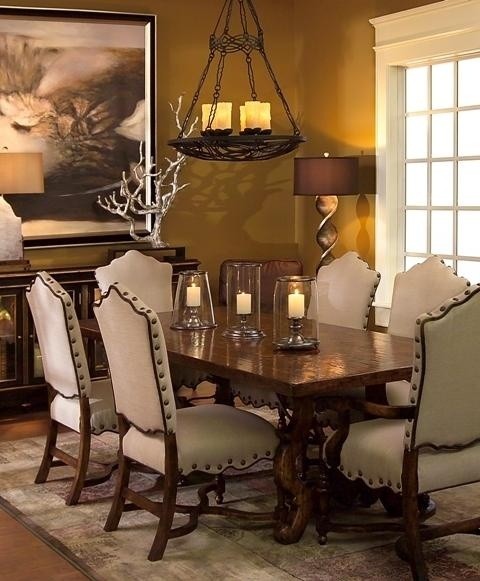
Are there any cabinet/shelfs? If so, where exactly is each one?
[0,260,202,417]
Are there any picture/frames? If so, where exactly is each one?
[0,5,160,250]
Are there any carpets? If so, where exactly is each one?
[0,403,480,581]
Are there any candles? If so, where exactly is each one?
[288,290,305,318]
[236,292,251,314]
[201,102,233,130]
[186,283,200,306]
[239,100,271,131]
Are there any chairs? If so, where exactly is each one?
[94,250,173,312]
[311,255,471,519]
[313,282,480,581]
[91,282,281,562]
[24,271,178,506]
[277,251,381,441]
[219,259,302,315]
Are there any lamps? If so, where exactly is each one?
[294,151,358,280]
[166,0,308,162]
[1,152,43,194]
